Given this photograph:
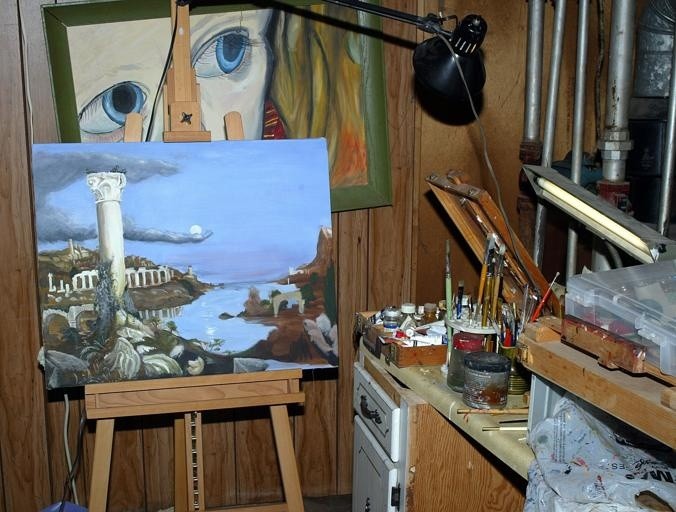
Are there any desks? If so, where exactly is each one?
[351,327,532,512]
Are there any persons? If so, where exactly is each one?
[66,2,276,144]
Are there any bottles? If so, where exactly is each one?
[383,302,436,332]
[447,332,511,411]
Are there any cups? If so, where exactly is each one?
[497,340,527,395]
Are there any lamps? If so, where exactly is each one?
[523,164,676,263]
[178,0,485,100]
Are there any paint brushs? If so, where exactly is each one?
[446,232,507,368]
[502,271,560,347]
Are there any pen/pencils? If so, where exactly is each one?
[482,427,528,431]
[499,419,528,423]
